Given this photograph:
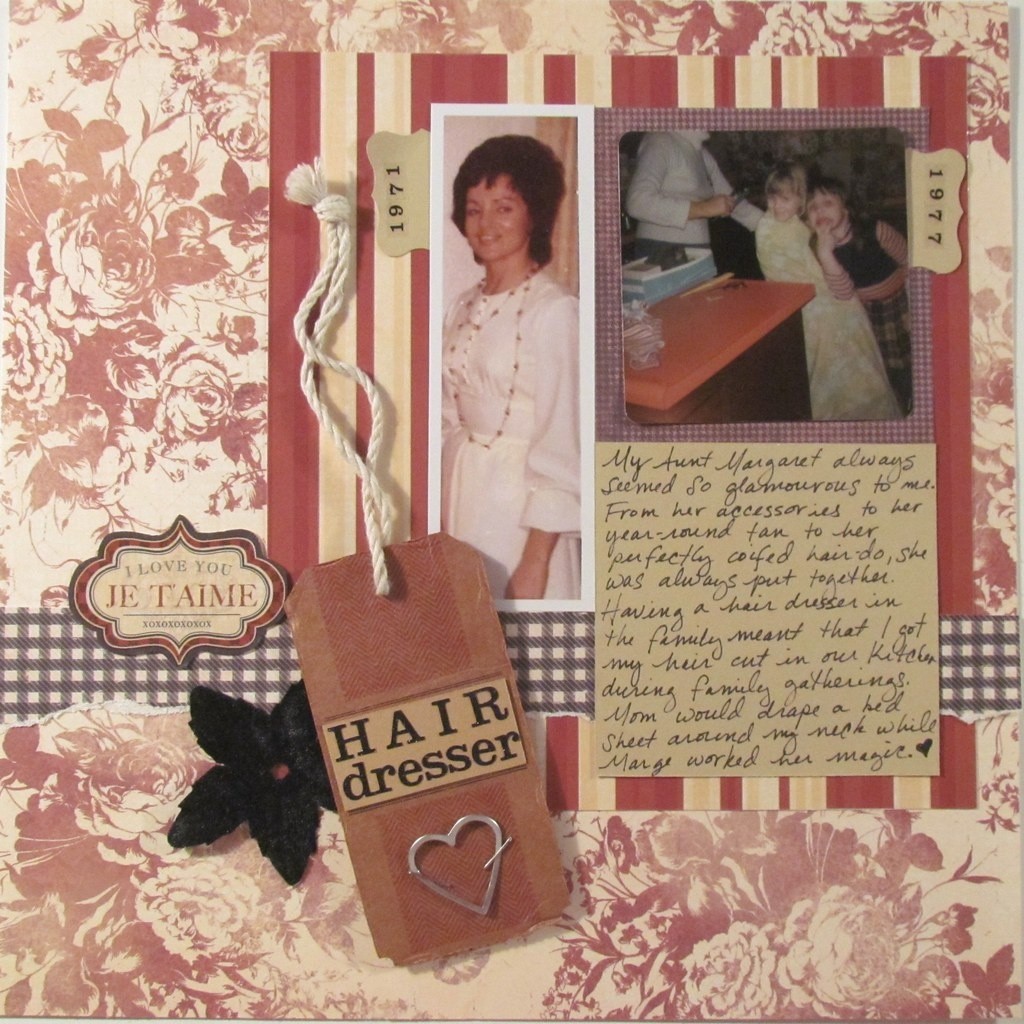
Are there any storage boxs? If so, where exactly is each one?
[621,247,717,307]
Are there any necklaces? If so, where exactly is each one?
[447,262,543,449]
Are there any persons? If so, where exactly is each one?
[625,130,767,261]
[754,161,903,421]
[440,133,579,598]
[799,176,907,416]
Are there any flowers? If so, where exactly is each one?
[168,680,339,886]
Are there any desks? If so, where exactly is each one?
[621,277,818,426]
